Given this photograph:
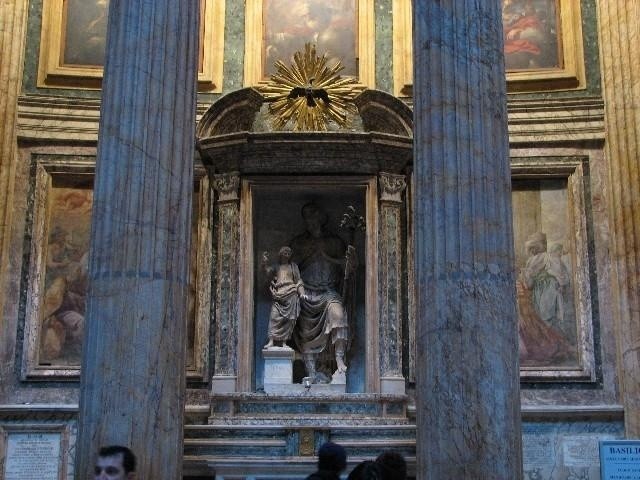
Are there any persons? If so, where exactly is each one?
[290,204,356,380]
[184,464,216,480]
[94,446,135,480]
[64,0,110,66]
[515,234,578,364]
[304,443,416,480]
[262,247,308,348]
[266,0,355,76]
[40,226,86,357]
[502,0,547,68]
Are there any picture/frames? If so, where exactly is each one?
[46,0,215,87]
[599,440,640,480]
[400,153,604,389]
[502,0,564,73]
[254,0,369,90]
[19,153,213,382]
